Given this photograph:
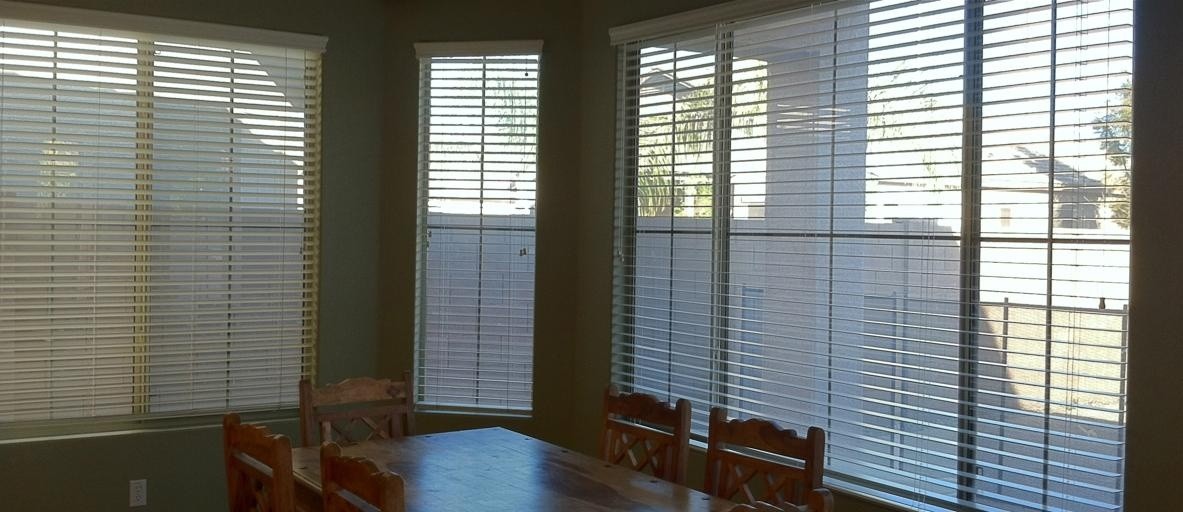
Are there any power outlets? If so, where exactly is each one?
[129,478,147,507]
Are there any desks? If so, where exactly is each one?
[294,426,778,511]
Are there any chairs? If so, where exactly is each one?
[728,488,833,510]
[299,369,415,449]
[223,411,295,510]
[601,382,692,486]
[704,404,824,503]
[319,440,405,510]
